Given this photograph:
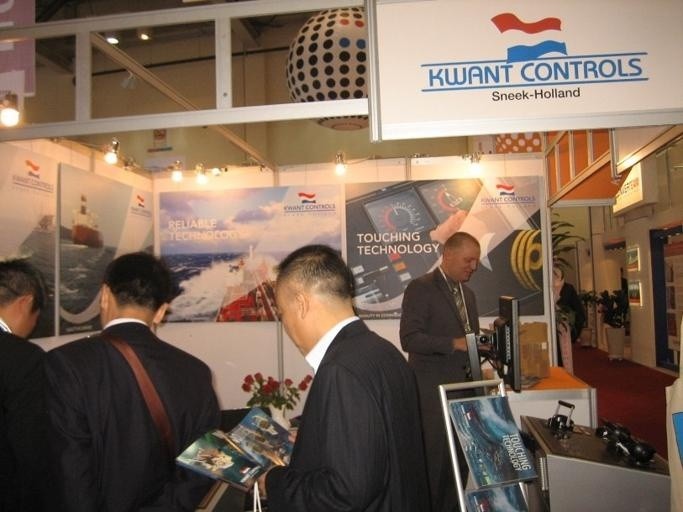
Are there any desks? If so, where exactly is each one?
[484,366,597,441]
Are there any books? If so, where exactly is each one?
[175,407,297,492]
[465,482,531,511]
[447,396,537,488]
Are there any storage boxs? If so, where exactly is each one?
[518,321,550,380]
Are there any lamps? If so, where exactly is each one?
[104,31,123,46]
[1,93,22,130]
[193,162,207,185]
[103,136,123,166]
[170,160,184,181]
[136,27,153,42]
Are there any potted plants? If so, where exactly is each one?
[597,288,629,363]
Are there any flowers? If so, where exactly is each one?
[241,372,314,415]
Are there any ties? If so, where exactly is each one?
[451,285,469,334]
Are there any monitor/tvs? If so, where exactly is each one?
[493,296,521,392]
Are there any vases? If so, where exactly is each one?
[266,402,293,433]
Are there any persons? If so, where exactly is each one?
[43,250,221,512]
[258,244,432,511]
[0,260,47,511]
[399,233,495,512]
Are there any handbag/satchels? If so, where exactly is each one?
[90,334,171,440]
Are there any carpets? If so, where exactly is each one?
[571,339,680,462]
